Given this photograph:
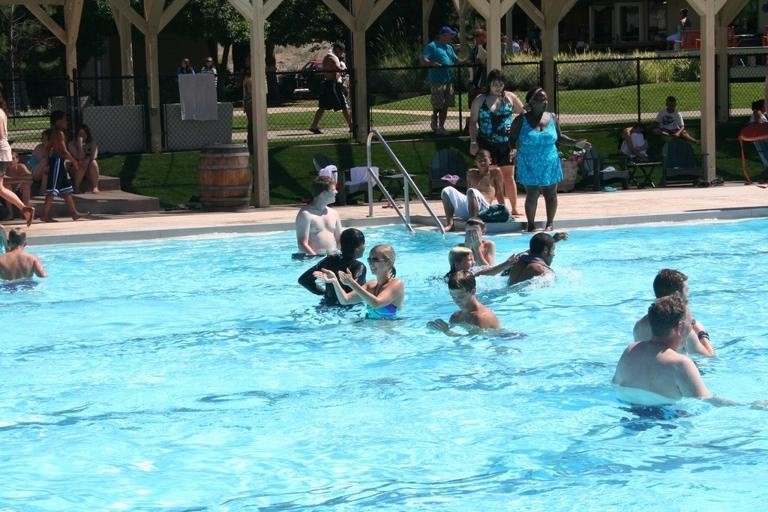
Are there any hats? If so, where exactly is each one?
[436,26,457,39]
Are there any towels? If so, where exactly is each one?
[317,165,339,184]
[440,174,460,185]
[343,166,380,187]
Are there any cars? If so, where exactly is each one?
[681,34,766,82]
[291,60,326,99]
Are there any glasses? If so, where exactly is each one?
[367,257,387,262]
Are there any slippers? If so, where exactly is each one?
[602,186,617,191]
[603,165,615,174]
[382,201,404,208]
[164,203,190,209]
[309,128,323,134]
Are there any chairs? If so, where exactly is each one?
[428,150,467,200]
[662,139,709,188]
[312,153,339,177]
[615,129,662,188]
[574,142,632,190]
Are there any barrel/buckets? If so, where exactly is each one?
[197,143,253,210]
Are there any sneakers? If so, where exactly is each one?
[692,177,710,187]
[711,177,724,186]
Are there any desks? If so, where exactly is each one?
[379,173,416,201]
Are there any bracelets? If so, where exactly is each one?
[697,330,711,341]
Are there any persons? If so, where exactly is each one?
[608,296,764,409]
[470,69,527,215]
[652,95,699,141]
[450,218,569,284]
[508,87,592,231]
[679,8,692,30]
[500,33,529,54]
[421,27,486,129]
[618,121,652,160]
[1,227,49,281]
[173,56,195,74]
[426,270,501,332]
[242,64,253,146]
[748,101,767,183]
[295,178,405,314]
[1,97,102,227]
[631,267,716,354]
[309,42,352,134]
[441,150,505,232]
[201,56,219,91]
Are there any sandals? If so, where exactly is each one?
[21,207,35,226]
